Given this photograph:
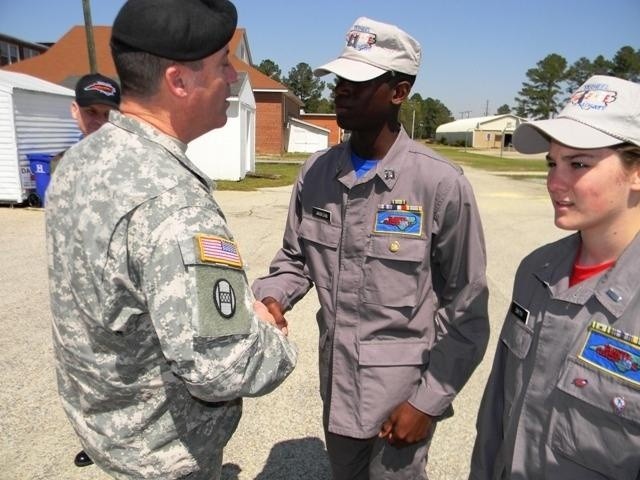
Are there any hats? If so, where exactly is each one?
[510,73,640,156]
[75,73,122,107]
[111,1,238,62]
[309,15,421,85]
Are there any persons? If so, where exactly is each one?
[468,74,640,480]
[249,16,491,480]
[49,72,121,466]
[45,0,300,480]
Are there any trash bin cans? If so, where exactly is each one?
[26,154,55,207]
